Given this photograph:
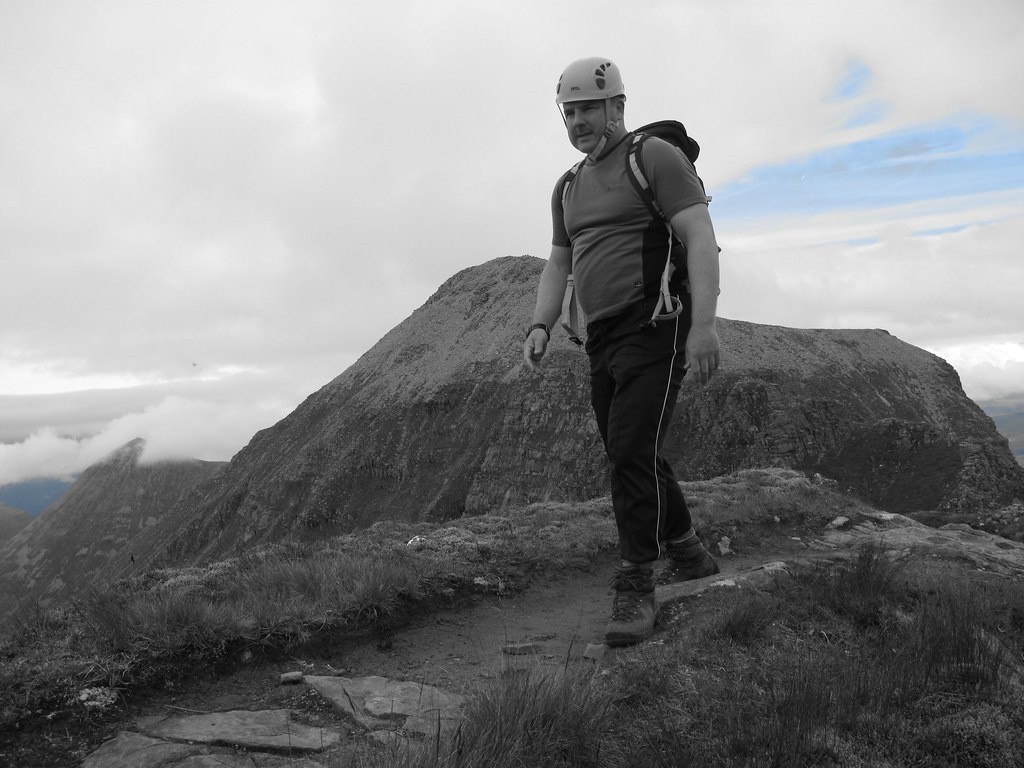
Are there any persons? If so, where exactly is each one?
[521,56,722,648]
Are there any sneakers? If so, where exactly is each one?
[605,564,661,647]
[661,526,720,583]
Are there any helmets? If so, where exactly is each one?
[556,57,627,102]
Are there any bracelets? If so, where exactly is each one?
[526,323,551,343]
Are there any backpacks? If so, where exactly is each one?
[561,120,721,346]
